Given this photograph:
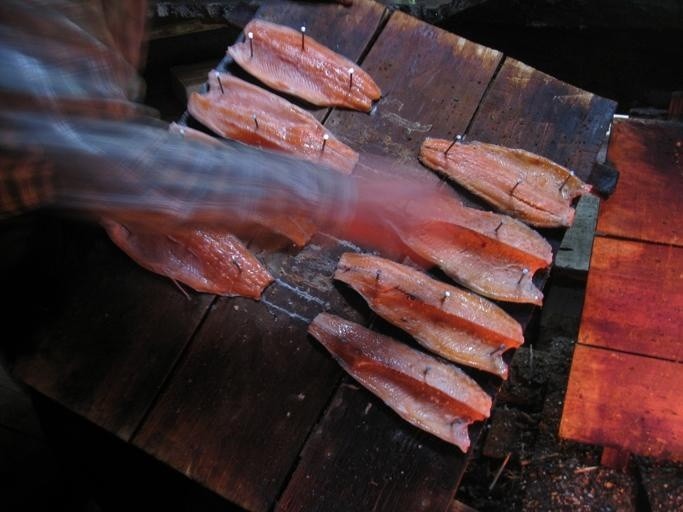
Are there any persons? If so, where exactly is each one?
[0,0,464,268]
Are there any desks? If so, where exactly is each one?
[559,117,683,473]
